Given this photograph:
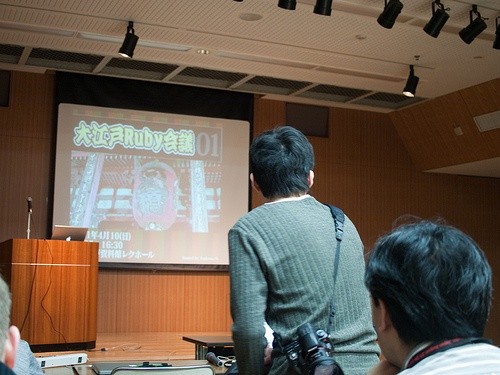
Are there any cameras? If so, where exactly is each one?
[285,322,345,375]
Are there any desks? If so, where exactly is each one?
[42,359,229,375]
[183,335,236,361]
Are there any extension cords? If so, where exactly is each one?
[36,352,89,369]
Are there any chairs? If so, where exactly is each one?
[113,363,217,375]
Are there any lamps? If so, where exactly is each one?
[312,0,333,17]
[402,63,424,100]
[458,4,488,45]
[277,0,297,11]
[492,16,500,50]
[376,0,404,30]
[119,20,140,58]
[422,0,450,38]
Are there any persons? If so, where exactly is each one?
[0,278,44,374]
[227,127,380,375]
[365,220,500,375]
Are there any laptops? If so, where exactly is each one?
[92,362,167,375]
[51,225,88,242]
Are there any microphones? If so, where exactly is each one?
[27,197,32,214]
[206,351,223,368]
